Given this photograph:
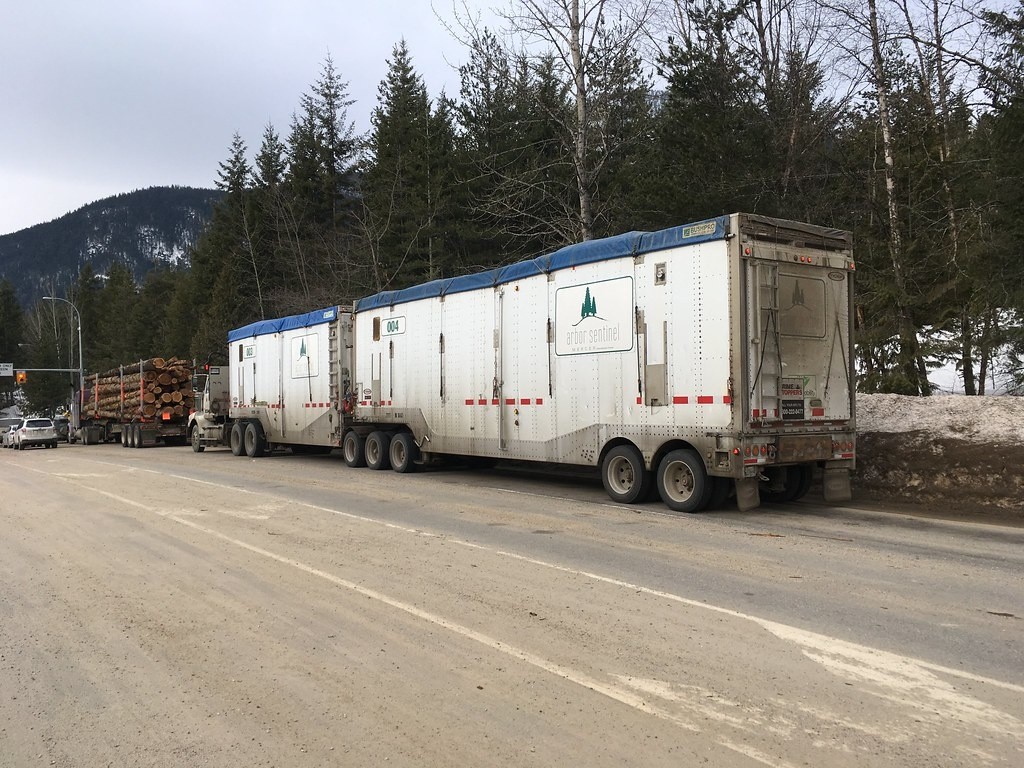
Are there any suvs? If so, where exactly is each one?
[12,416,58,450]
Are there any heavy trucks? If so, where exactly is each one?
[65,386,204,449]
[186,213,856,514]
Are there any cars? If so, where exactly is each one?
[1,415,73,450]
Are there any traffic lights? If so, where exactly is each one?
[16,370,25,383]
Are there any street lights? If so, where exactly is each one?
[42,296,84,390]
